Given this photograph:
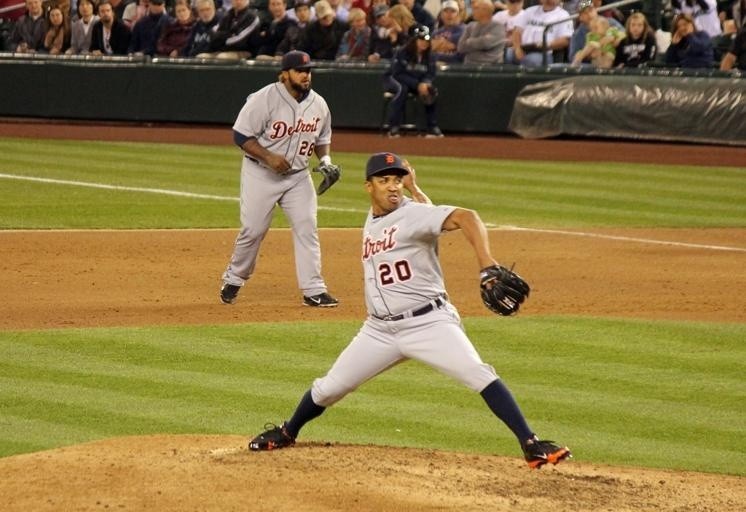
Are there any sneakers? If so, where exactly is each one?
[302,293,339,307]
[524,433,572,470]
[219,282,240,304]
[249,421,295,451]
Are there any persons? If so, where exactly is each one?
[0,1,746,71]
[218,48,341,308]
[382,25,445,139]
[247,151,572,471]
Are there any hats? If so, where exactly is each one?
[442,1,460,10]
[413,25,431,41]
[315,0,334,19]
[365,152,409,178]
[281,49,319,69]
[373,5,391,18]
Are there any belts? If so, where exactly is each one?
[371,296,447,321]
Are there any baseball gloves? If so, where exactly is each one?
[479,263,529,316]
[311,160,341,195]
